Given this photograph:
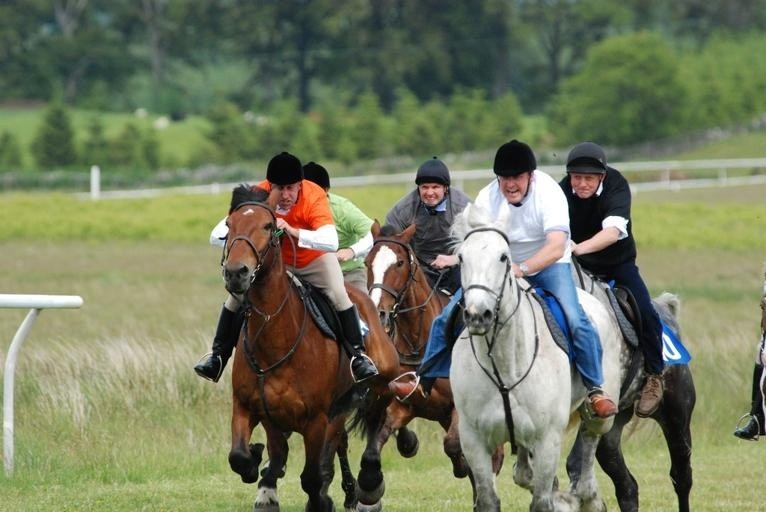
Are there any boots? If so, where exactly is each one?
[193,306,240,383]
[738,361,766,439]
[339,304,378,383]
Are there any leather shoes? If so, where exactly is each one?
[637,373,668,416]
[388,379,430,408]
[588,392,619,418]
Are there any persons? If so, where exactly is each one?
[732,273,766,439]
[557,141,665,419]
[194,152,380,383]
[381,156,475,296]
[388,139,620,418]
[303,161,376,297]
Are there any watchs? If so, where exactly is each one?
[519,262,530,276]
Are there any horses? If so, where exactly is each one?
[358,218,468,480]
[254,410,359,512]
[569,253,695,512]
[221,183,423,511]
[449,204,624,512]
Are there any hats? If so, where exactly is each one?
[265,152,304,186]
[492,140,538,176]
[414,158,451,186]
[302,162,329,192]
[566,142,609,172]
